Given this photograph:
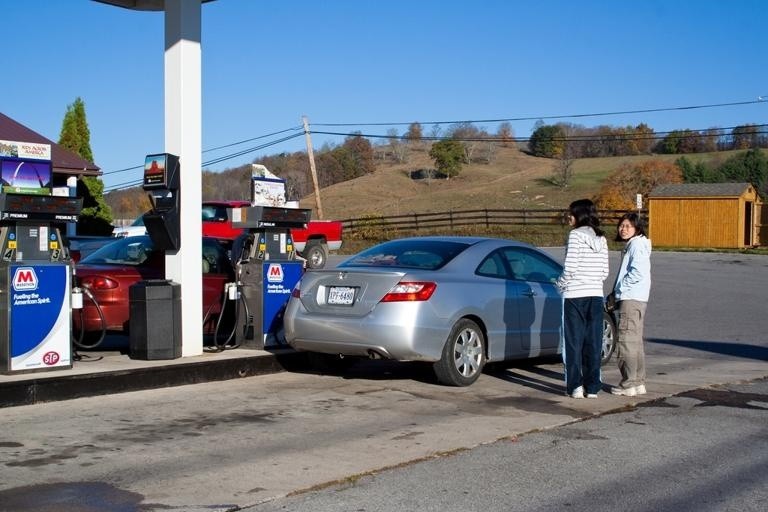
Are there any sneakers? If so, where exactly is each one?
[611,384,646,396]
[565,386,584,399]
[586,392,598,399]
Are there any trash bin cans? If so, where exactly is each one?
[128,282,182,360]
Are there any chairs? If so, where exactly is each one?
[509,259,527,280]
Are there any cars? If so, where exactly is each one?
[73,234,230,339]
[283,236,618,385]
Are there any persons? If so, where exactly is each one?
[549,197,609,399]
[604,212,653,396]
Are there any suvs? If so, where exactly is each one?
[111,208,155,238]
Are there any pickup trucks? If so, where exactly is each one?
[198,197,344,271]
